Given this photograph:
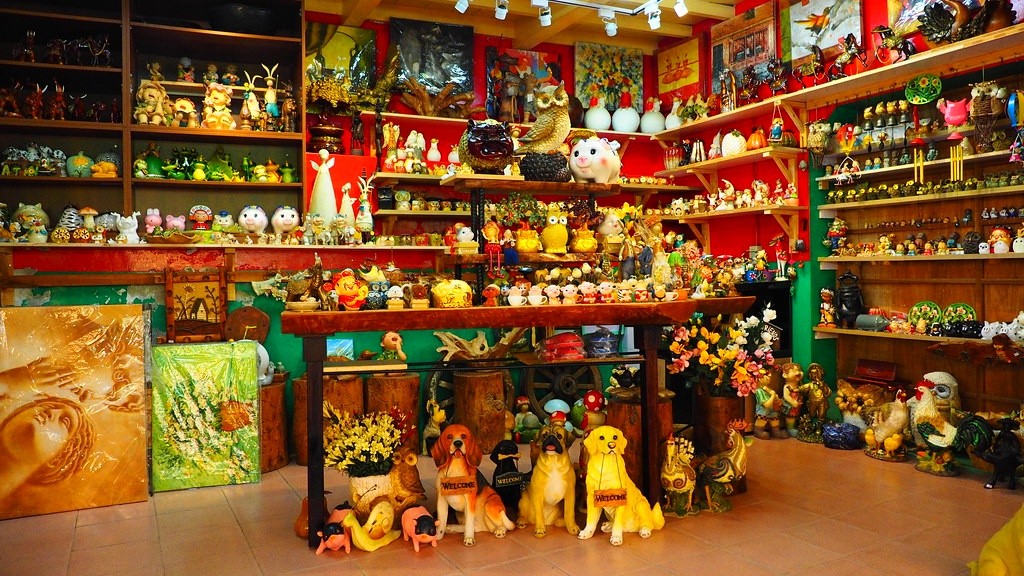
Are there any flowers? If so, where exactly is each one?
[319,400,417,478]
[306,78,350,115]
[667,301,783,398]
[757,249,768,272]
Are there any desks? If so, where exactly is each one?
[280,293,757,551]
[732,280,792,362]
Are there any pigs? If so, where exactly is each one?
[507,282,616,306]
[238,205,298,234]
[166,215,186,231]
[569,135,622,184]
[316,500,355,554]
[401,504,440,553]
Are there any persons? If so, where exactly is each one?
[863,209,972,255]
[376,331,406,360]
[752,362,832,441]
[824,149,912,185]
[818,288,836,328]
[133,57,241,130]
[707,178,782,208]
[499,229,519,271]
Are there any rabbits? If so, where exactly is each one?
[145,208,163,234]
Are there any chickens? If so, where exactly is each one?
[659,420,748,512]
[862,388,993,476]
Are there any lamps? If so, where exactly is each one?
[597,8,619,37]
[834,270,869,328]
[454,0,469,13]
[494,0,509,21]
[644,2,661,29]
[673,0,689,18]
[531,0,552,26]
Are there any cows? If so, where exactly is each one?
[0,80,107,121]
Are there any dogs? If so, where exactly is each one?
[431,424,665,547]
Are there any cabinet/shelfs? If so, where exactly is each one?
[0,0,1024,416]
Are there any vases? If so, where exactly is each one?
[697,392,747,496]
[348,473,390,509]
[762,270,773,282]
